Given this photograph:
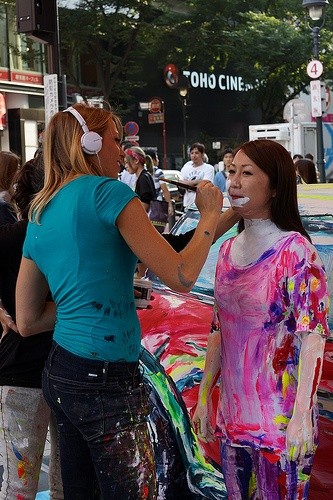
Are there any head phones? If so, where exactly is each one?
[63,106,103,155]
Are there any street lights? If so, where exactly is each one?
[301,0,330,184]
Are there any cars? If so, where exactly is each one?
[160,170,184,202]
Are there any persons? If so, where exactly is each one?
[193,140,330,500]
[15,102,224,500]
[0,141,318,500]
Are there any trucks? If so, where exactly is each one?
[249,122,333,184]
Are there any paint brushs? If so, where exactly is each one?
[159,175,198,192]
